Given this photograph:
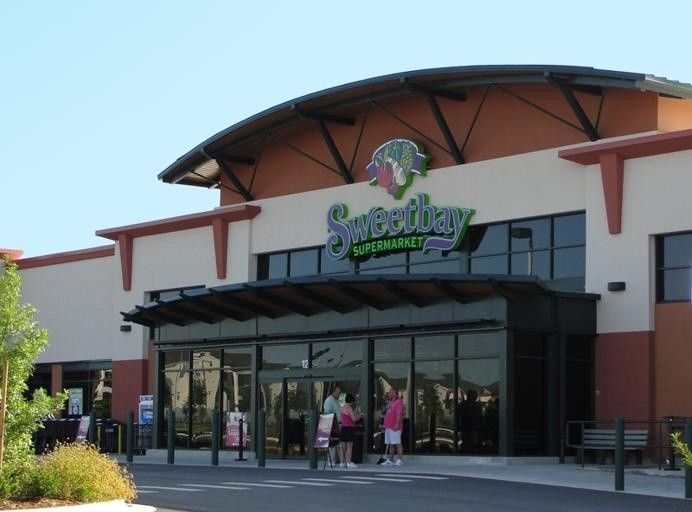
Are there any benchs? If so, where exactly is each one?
[572,429,649,465]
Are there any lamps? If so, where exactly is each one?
[120,325,131,331]
[608,282,625,291]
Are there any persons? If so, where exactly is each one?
[323,387,342,467]
[340,393,361,467]
[382,387,405,465]
[456,389,483,456]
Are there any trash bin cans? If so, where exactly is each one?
[33,418,80,455]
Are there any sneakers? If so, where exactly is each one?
[381,458,403,465]
[329,462,359,469]
